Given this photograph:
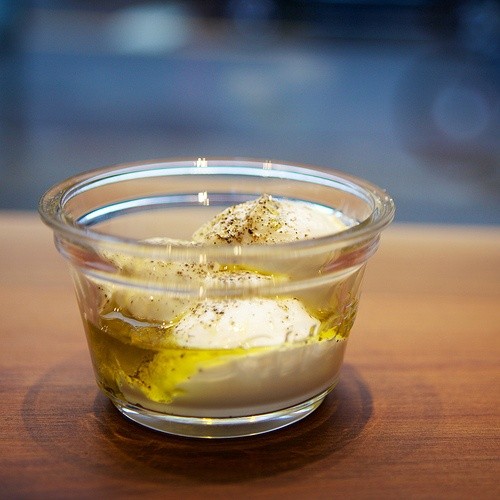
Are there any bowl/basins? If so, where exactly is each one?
[38,155,396,439]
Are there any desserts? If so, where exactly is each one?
[86,193,352,406]
